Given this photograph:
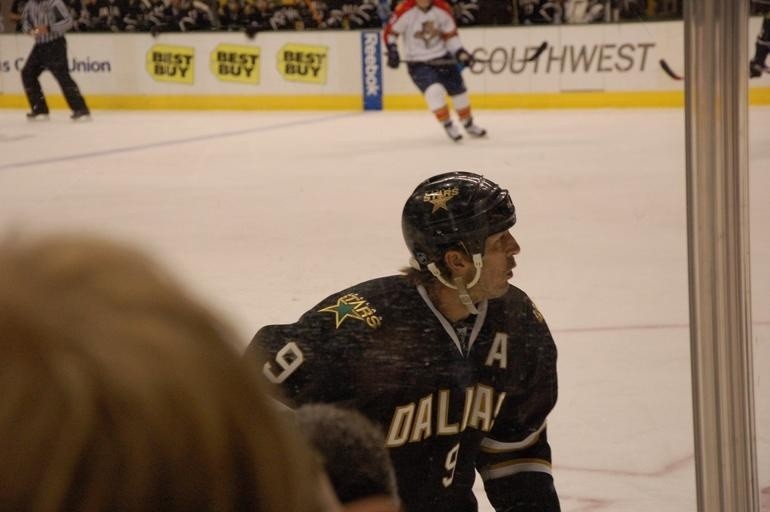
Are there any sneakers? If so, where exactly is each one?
[72,108,87,118]
[445,122,462,140]
[26,109,48,118]
[466,120,485,136]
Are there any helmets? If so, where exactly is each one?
[401,172,516,266]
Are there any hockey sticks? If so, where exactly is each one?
[660,60,683,82]
[398,41,547,64]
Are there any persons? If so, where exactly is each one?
[749,11,770,77]
[0,1,685,32]
[295,401,403,512]
[21,0,90,120]
[241,171,561,512]
[383,0,486,141]
[0,230,322,512]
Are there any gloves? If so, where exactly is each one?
[387,45,399,68]
[456,51,473,67]
